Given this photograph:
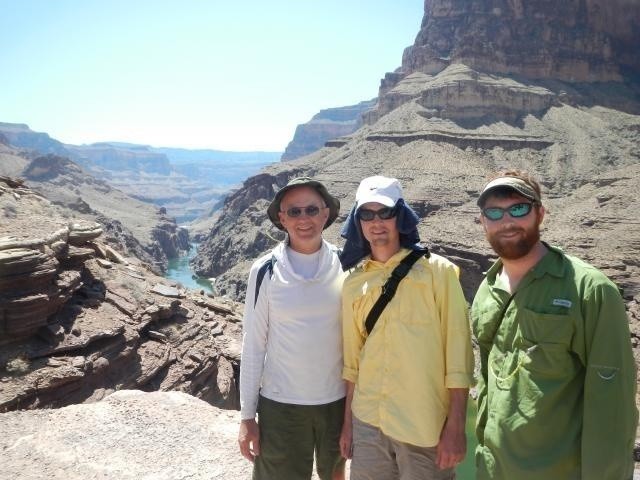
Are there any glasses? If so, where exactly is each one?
[480,201,536,221]
[279,205,327,218]
[357,209,399,220]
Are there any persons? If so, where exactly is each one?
[338,175,480,480]
[469,172,639,480]
[237,176,365,480]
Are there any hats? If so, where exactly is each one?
[477,173,540,208]
[267,176,340,233]
[356,176,403,210]
